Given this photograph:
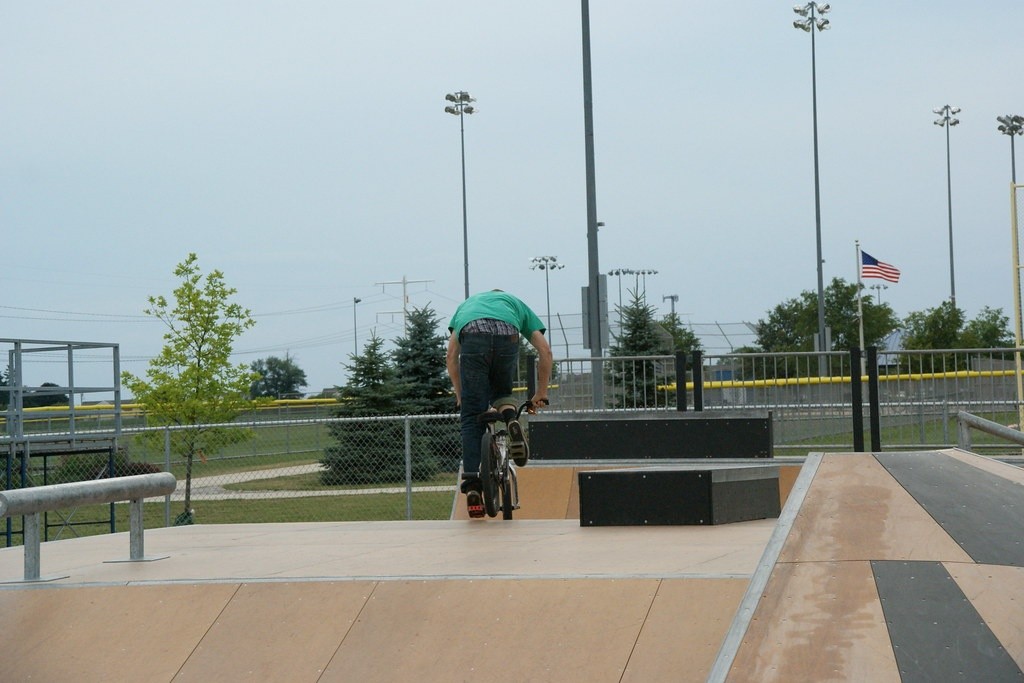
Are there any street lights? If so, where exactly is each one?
[635,270,658,305]
[870,284,888,305]
[998,114,1024,183]
[934,105,961,306]
[354,297,361,357]
[609,269,635,336]
[529,256,564,349]
[793,1,832,352]
[445,90,476,299]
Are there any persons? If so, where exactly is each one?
[447,289,553,518]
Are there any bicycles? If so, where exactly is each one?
[454,399,549,520]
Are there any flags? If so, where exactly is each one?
[861,251,900,283]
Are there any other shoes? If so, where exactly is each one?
[464,487,486,519]
[507,417,530,468]
